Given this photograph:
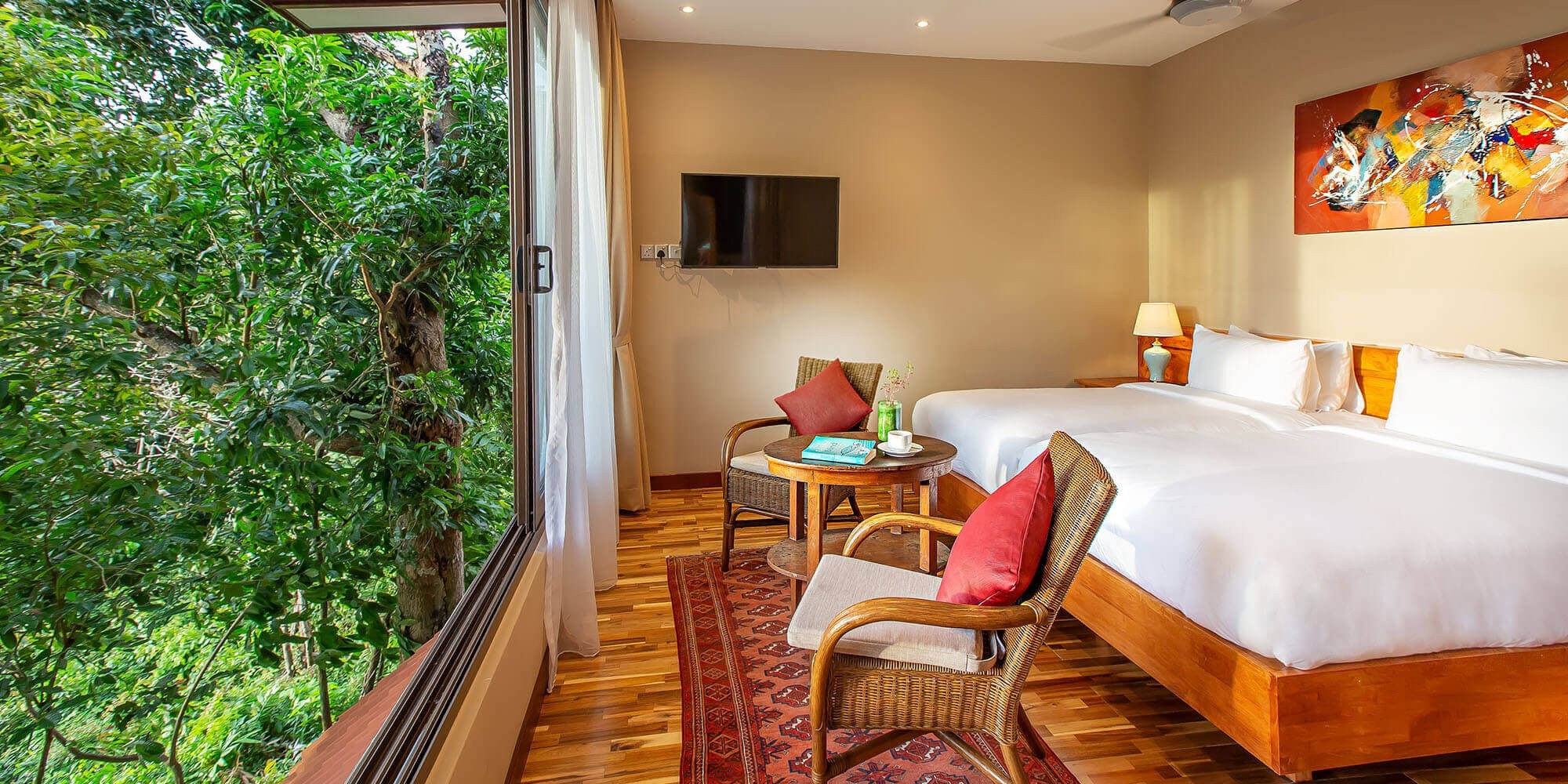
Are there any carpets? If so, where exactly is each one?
[665,544,1082,784]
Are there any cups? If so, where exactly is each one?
[877,400,902,442]
[887,430,912,451]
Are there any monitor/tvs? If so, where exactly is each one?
[680,173,841,268]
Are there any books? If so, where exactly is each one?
[802,436,877,465]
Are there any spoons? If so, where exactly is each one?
[911,447,924,450]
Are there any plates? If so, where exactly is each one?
[876,442,923,457]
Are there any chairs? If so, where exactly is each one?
[719,355,883,573]
[809,429,1118,784]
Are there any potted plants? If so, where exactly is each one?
[877,359,915,442]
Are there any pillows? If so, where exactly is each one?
[1186,322,1322,413]
[1226,324,1352,411]
[1462,343,1567,368]
[1383,342,1568,469]
[936,447,1056,606]
[774,357,874,436]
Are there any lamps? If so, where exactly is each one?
[1132,302,1184,383]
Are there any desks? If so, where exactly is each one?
[761,431,958,682]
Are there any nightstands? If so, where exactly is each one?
[1074,376,1170,390]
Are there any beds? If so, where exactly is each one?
[911,381,1568,784]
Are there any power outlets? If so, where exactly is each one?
[640,244,655,259]
[654,244,669,260]
